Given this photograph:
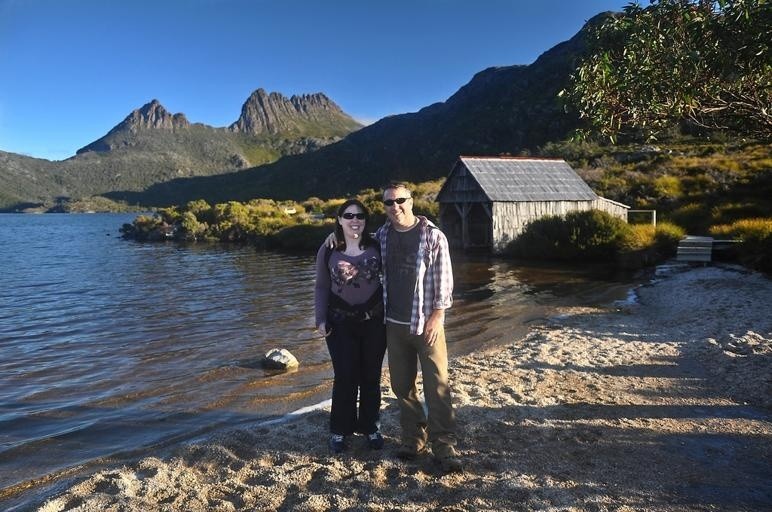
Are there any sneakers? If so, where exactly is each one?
[367,432,384,448]
[399,441,426,457]
[331,434,345,452]
[434,446,462,470]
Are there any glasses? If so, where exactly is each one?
[384,197,410,206]
[344,213,365,219]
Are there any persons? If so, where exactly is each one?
[314,198,387,453]
[324,182,462,474]
